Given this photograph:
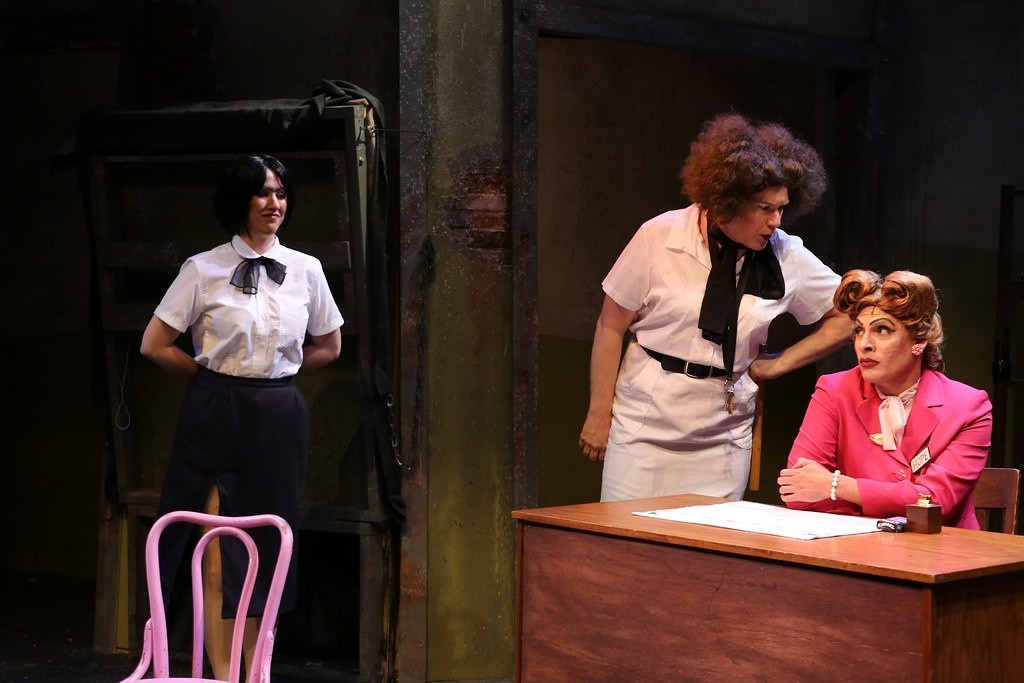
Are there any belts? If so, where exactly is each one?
[641,346,727,379]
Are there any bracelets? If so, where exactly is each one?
[830,469,841,502]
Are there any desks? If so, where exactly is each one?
[510,494,1024,683]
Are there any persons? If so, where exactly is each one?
[579,115,858,502]
[138,153,345,683]
[777,267,993,538]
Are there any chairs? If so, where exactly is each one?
[116,510,294,683]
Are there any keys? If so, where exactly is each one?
[722,379,736,415]
